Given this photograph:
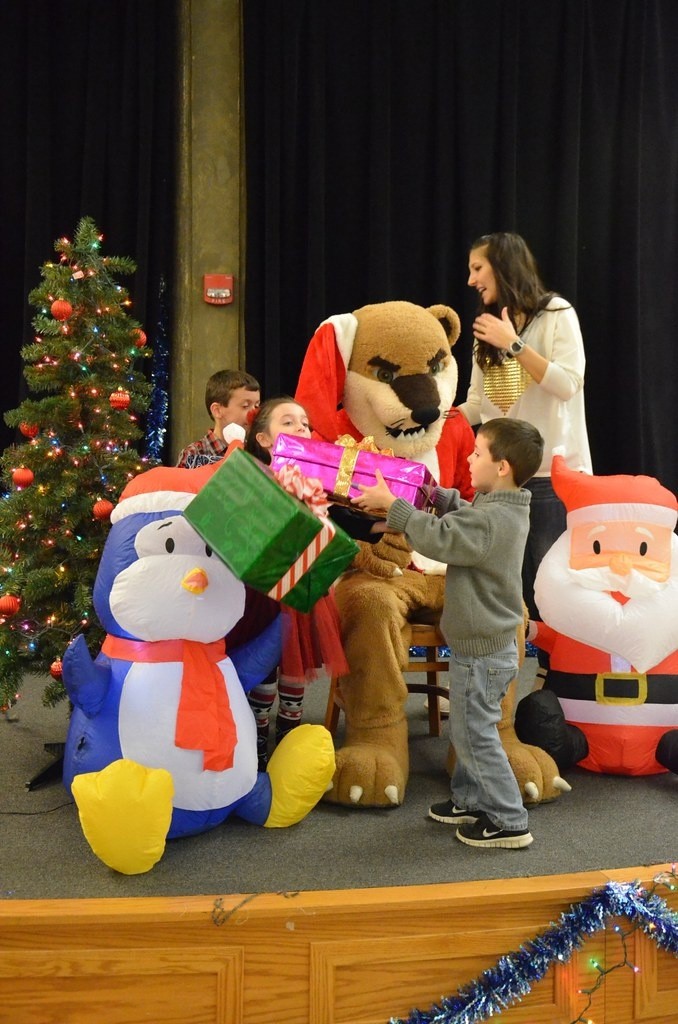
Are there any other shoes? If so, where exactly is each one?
[257,750,270,773]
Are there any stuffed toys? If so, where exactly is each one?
[310,301,572,806]
[515,445,678,776]
[62,466,338,876]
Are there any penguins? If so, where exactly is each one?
[58,511,338,876]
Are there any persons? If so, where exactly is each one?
[349,418,543,848]
[245,399,403,773]
[175,370,260,468]
[455,231,595,691]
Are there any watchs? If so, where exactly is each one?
[505,340,526,359]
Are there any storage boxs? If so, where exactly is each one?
[270,432,439,520]
[181,445,361,614]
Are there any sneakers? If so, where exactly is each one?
[455,814,534,849]
[428,798,484,826]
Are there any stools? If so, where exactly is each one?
[322,623,450,736]
